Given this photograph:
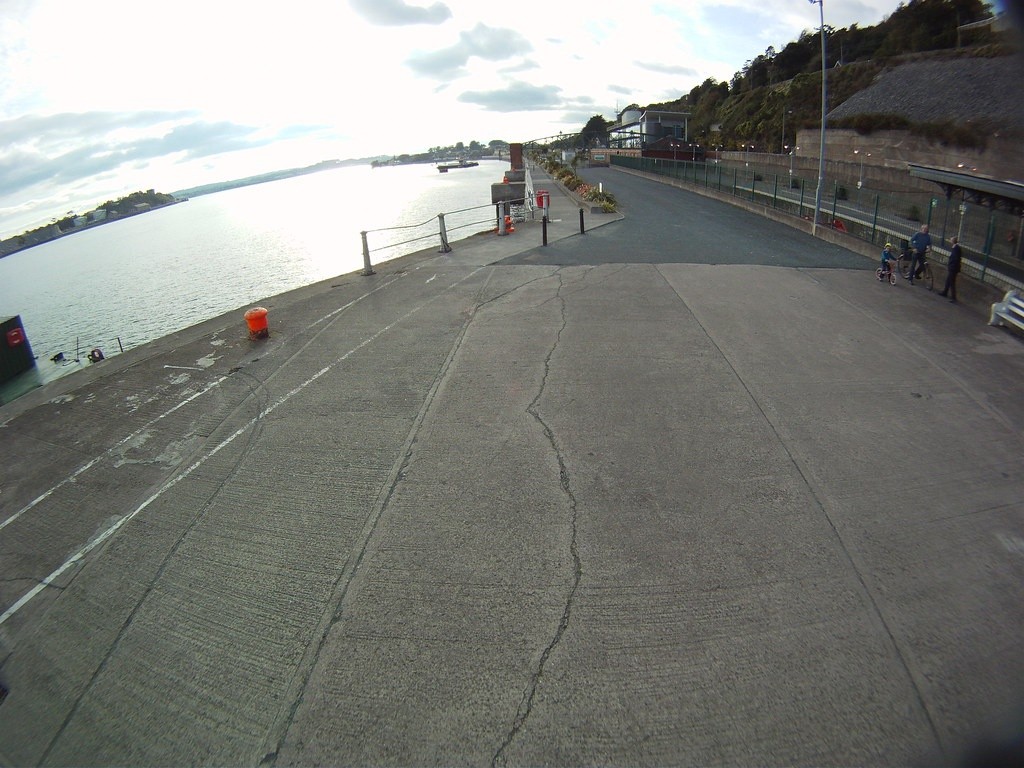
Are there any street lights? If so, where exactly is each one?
[741,144,754,181]
[559,131,562,168]
[670,143,680,168]
[783,144,800,190]
[712,144,723,173]
[688,144,700,167]
[853,149,872,188]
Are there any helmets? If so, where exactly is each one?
[884,243,891,247]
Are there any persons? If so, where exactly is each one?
[909,224,932,284]
[879,243,899,281]
[938,236,962,302]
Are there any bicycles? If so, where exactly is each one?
[877,268,897,286]
[899,257,934,291]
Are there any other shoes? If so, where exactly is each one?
[879,276,883,281]
[938,292,947,297]
[909,279,913,285]
[913,272,922,279]
[949,297,957,303]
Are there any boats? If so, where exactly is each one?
[436,156,478,168]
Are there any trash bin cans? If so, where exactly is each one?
[536,190,550,208]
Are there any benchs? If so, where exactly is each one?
[988,290,1024,329]
